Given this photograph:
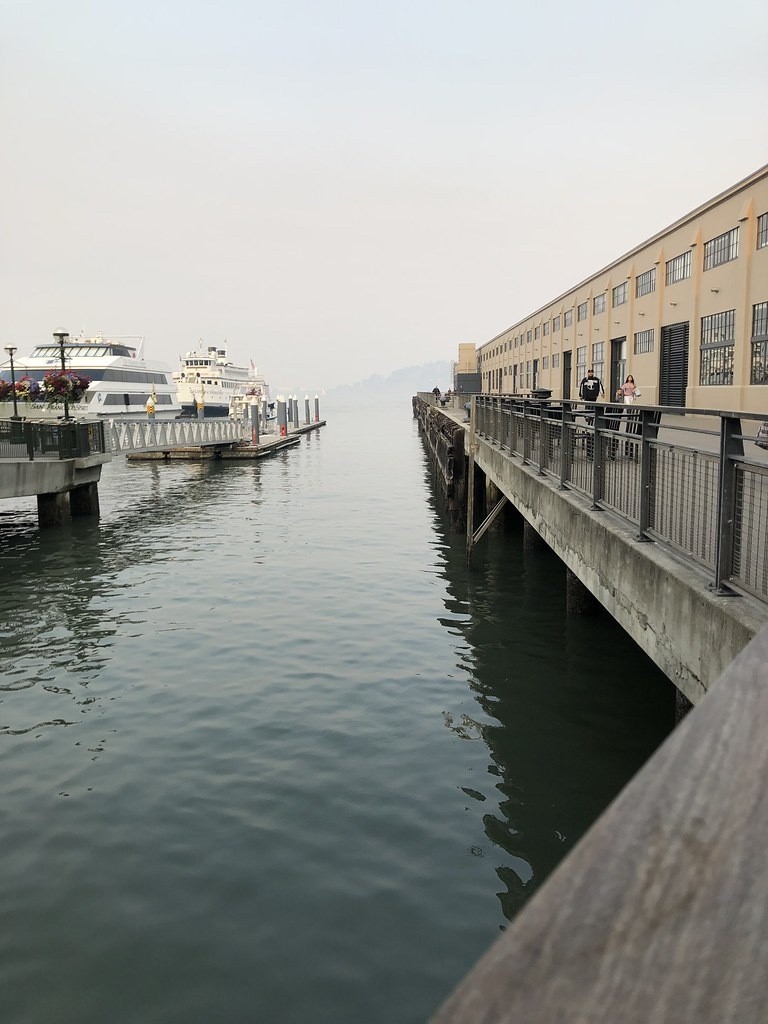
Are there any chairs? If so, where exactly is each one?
[503,398,662,462]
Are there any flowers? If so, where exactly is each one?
[0,369,92,407]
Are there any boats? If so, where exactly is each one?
[0,329,182,423]
[171,336,271,418]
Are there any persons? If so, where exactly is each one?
[616,375,637,405]
[615,388,623,402]
[448,388,451,394]
[579,368,605,423]
[432,386,440,396]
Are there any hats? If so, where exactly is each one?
[588,369,593,373]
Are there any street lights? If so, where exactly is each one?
[52,327,75,422]
[3,343,24,421]
